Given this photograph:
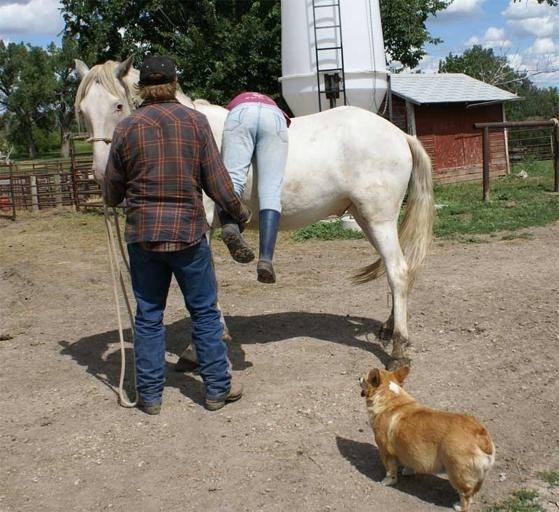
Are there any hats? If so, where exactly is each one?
[138,55,177,86]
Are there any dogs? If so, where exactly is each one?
[355,365,496,512]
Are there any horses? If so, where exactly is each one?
[72,54,436,373]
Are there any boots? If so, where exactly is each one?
[215,189,254,263]
[255,207,282,284]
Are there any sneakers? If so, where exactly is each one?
[136,397,163,417]
[203,381,243,412]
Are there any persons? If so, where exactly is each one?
[217,90,291,284]
[102,56,253,414]
